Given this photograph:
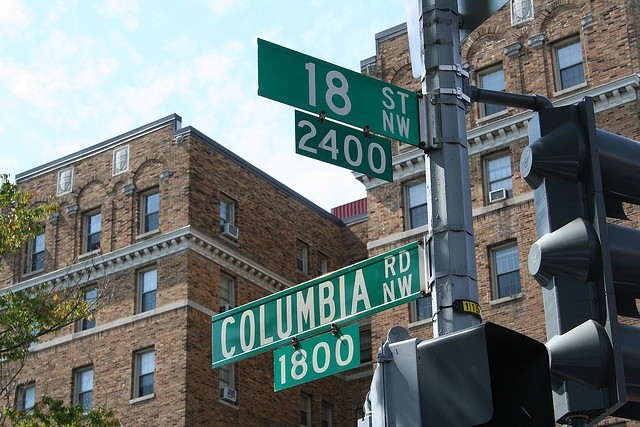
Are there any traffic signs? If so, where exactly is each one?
[211,237,429,369]
[294,108,394,183]
[256,37,420,147]
[272,321,361,393]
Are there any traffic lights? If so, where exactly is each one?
[519,95,639,426]
